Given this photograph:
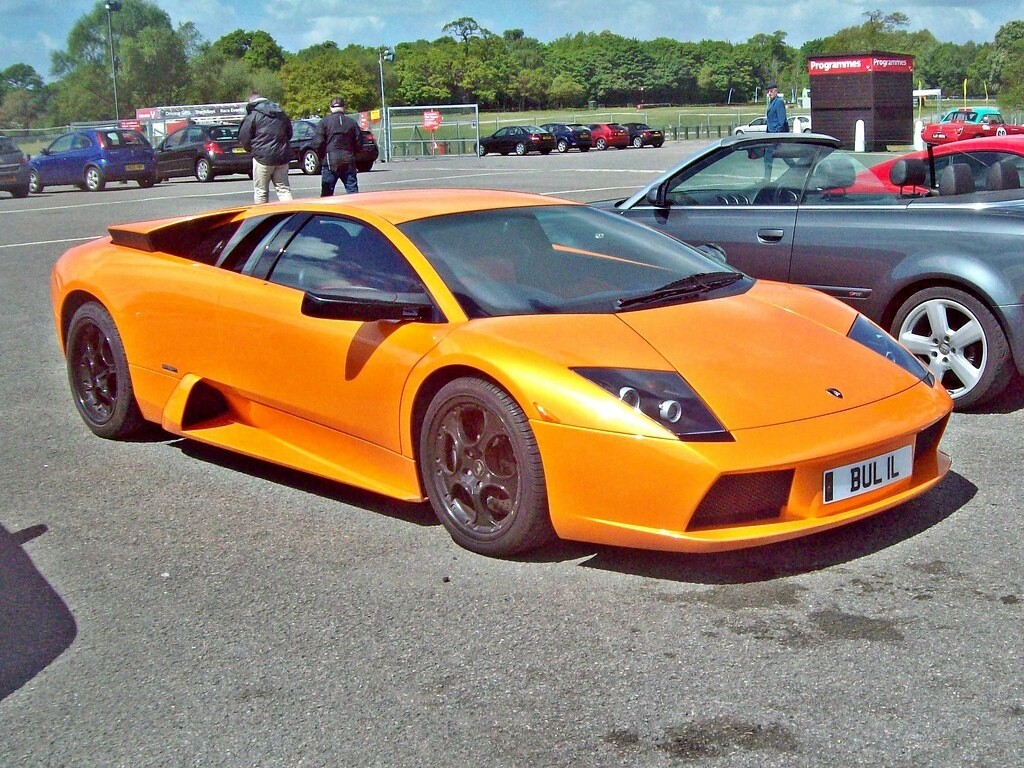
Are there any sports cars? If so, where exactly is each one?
[582,130,1023,409]
[52,188,957,560]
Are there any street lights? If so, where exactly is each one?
[104,0,125,121]
[377,46,397,163]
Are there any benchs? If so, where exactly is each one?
[939,161,1020,196]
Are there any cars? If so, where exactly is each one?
[474,121,665,155]
[284,118,380,174]
[921,106,1024,146]
[26,129,154,193]
[0,135,31,199]
[732,115,813,134]
[152,123,254,182]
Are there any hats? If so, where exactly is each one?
[246,92,262,105]
[765,84,778,90]
[331,94,345,111]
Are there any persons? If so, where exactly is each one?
[314,98,362,197]
[238,92,293,203]
[755,85,795,183]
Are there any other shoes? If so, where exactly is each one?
[755,178,769,184]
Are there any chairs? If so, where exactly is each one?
[889,159,926,199]
[807,158,856,202]
[77,138,90,148]
